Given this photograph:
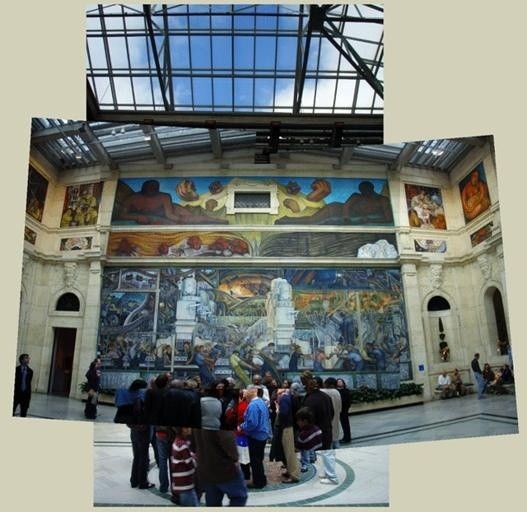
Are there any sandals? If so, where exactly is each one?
[282,472,298,483]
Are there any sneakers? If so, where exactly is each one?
[319,474,334,484]
[301,467,308,472]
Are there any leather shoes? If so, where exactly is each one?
[132,483,155,489]
[247,481,266,489]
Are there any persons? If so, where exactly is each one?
[12,352,34,417]
[471,352,487,400]
[114,371,355,508]
[485,366,503,394]
[450,368,462,398]
[84,361,102,419]
[93,357,102,406]
[495,363,513,385]
[482,362,494,393]
[459,169,491,245]
[438,369,454,400]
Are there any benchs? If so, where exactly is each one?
[486,381,515,396]
[434,382,475,399]
